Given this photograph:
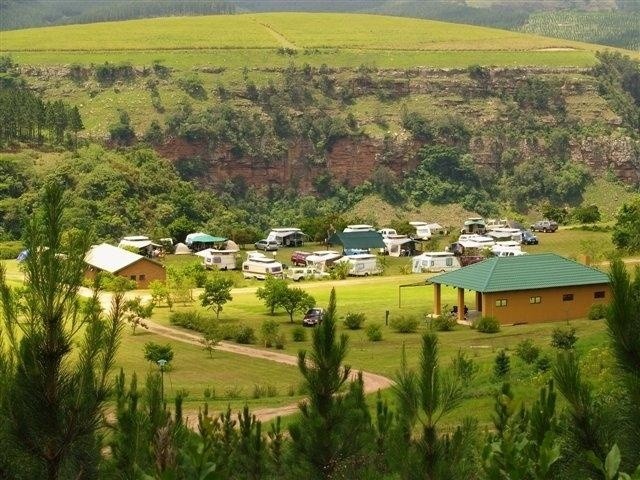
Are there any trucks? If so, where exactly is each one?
[343,224,423,258]
[267,228,305,247]
[408,222,432,242]
[445,218,525,257]
[411,251,462,273]
[204,249,237,271]
[241,257,284,280]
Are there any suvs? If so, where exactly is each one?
[291,251,313,266]
[530,221,558,233]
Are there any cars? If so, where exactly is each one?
[247,252,266,260]
[303,308,327,327]
[522,230,538,245]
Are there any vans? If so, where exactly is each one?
[254,239,278,251]
[336,254,384,277]
[306,251,339,266]
[292,268,315,282]
